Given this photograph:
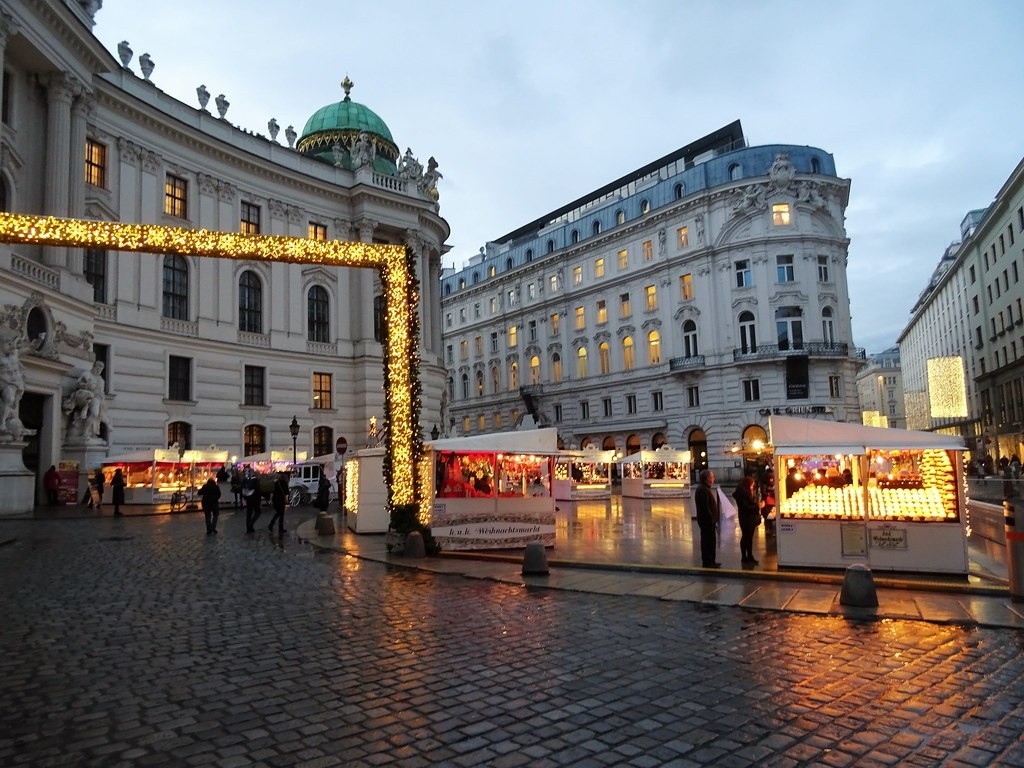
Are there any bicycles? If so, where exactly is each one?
[169,489,188,513]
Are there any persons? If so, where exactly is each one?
[402,148,416,169]
[43,465,61,504]
[731,476,765,568]
[350,134,373,171]
[231,466,348,535]
[62,361,113,438]
[967,453,1022,485]
[87,469,105,509]
[473,475,491,494]
[695,470,722,568]
[733,185,768,216]
[796,181,827,208]
[197,478,221,535]
[744,459,853,517]
[0,327,45,430]
[110,469,127,516]
[216,466,230,483]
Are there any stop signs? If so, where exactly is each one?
[336,438,347,455]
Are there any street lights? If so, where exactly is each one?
[289,414,300,465]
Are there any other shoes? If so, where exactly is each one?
[747,555,758,566]
[741,559,754,570]
[702,562,721,568]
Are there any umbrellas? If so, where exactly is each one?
[715,489,737,550]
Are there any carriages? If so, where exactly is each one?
[230,460,336,509]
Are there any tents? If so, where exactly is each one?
[95,446,228,504]
[560,447,692,499]
[427,427,556,514]
[769,415,970,574]
[243,450,307,473]
